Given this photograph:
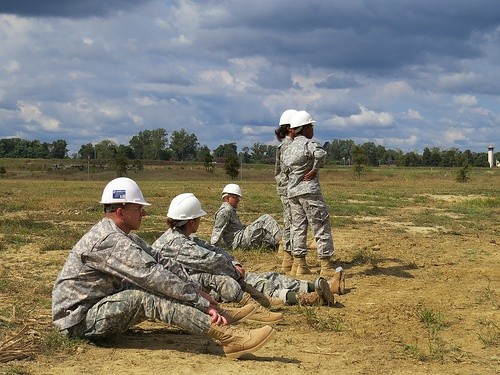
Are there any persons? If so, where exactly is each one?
[274,109,336,276]
[210,183,281,254]
[51,177,276,359]
[150,193,245,304]
[243,267,345,306]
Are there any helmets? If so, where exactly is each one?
[279,109,298,125]
[290,110,316,129]
[100,177,152,206]
[167,192,207,220]
[222,183,242,197]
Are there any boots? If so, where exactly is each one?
[278,243,284,256]
[320,259,336,277]
[240,280,284,310]
[208,321,276,359]
[236,292,285,324]
[282,250,294,271]
[297,257,319,280]
[214,302,255,325]
[290,255,298,276]
[328,267,346,295]
[299,277,334,306]
